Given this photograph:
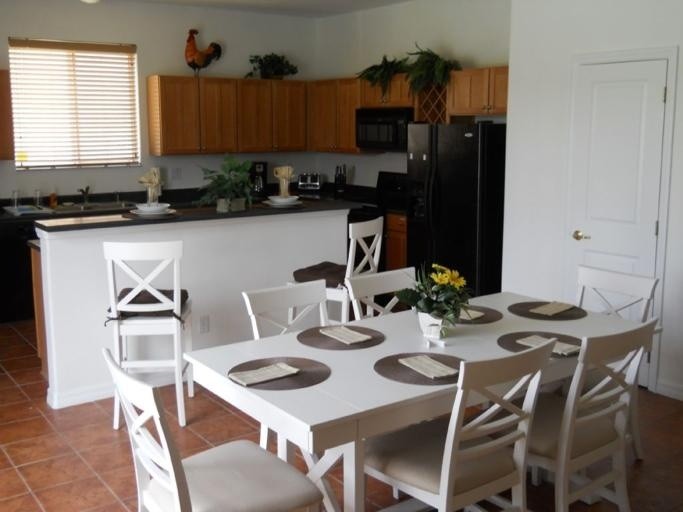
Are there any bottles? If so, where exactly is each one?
[334,164,347,194]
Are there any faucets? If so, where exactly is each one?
[77,185,91,201]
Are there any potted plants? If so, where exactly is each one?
[242,53,298,80]
[207,152,254,212]
[191,175,232,213]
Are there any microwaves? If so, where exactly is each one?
[354,107,414,151]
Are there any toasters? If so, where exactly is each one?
[298,174,322,192]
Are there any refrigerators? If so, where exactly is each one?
[407,121,505,300]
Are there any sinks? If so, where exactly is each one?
[53,201,135,213]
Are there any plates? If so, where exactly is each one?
[129,203,177,216]
[261,196,305,207]
